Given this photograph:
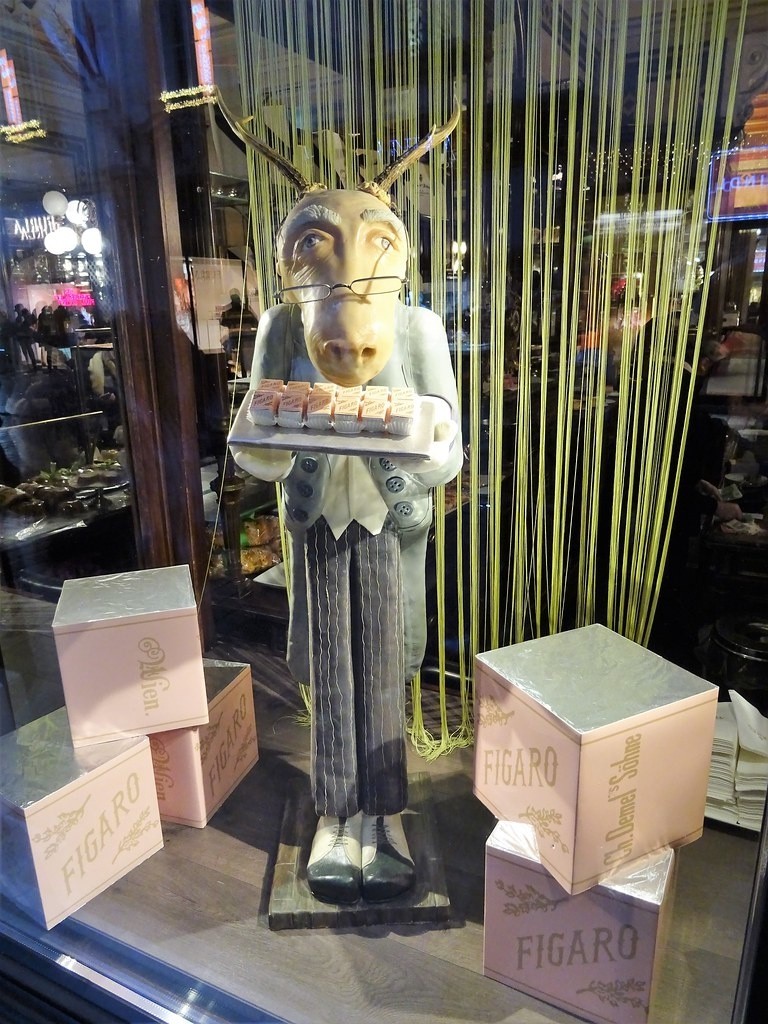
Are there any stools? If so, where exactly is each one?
[706,615,767,715]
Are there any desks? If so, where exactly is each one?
[0,484,138,590]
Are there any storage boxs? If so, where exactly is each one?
[473,623,720,896]
[52,563,210,749]
[483,820,676,1024]
[146,658,259,829]
[1,704,164,930]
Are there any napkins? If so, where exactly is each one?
[705,690,768,832]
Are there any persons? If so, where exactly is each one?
[605,314,758,651]
[10,303,71,366]
